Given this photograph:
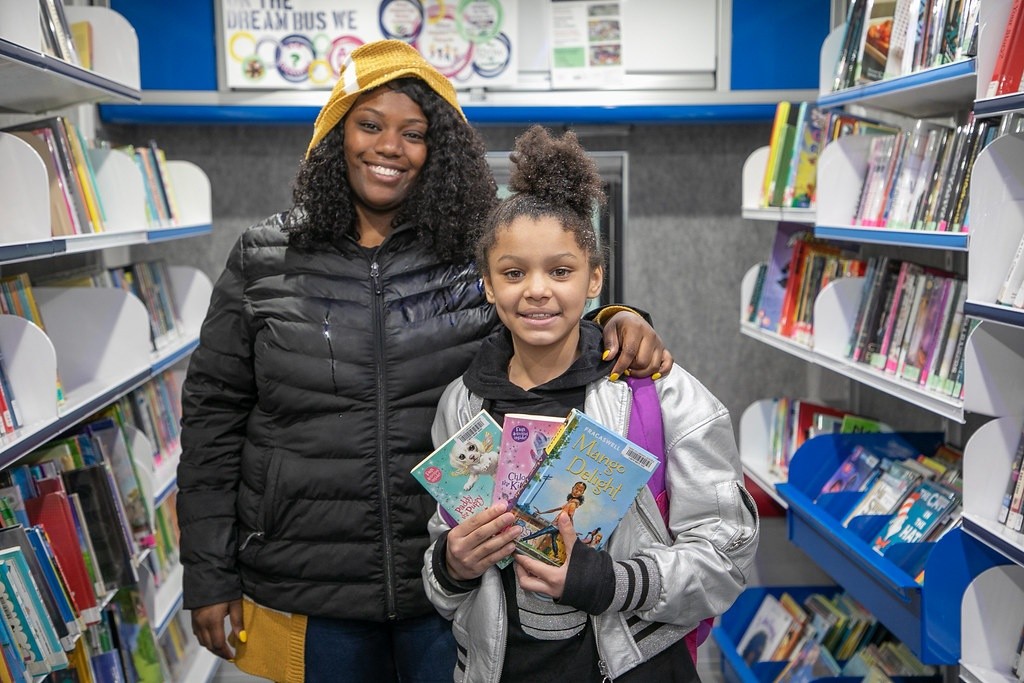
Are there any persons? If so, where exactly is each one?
[420,122,762,682]
[176,37,675,683]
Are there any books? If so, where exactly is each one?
[0,0,199,683]
[492,413,567,533]
[503,407,661,565]
[733,0,1024,683]
[409,408,515,570]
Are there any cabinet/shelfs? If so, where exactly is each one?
[97,0,848,121]
[708,1,1023,683]
[0,0,233,683]
